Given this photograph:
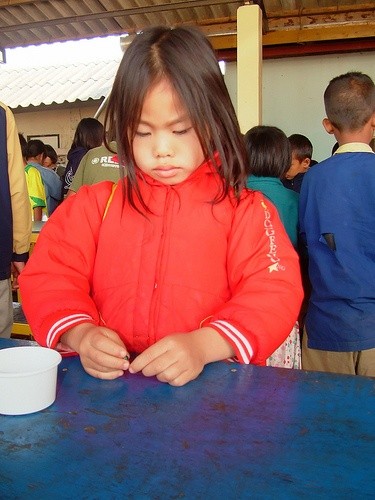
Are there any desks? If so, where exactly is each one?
[0,337,375,500]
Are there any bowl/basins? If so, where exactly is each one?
[0,347,63,415]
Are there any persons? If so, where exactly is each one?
[0,25,375,388]
